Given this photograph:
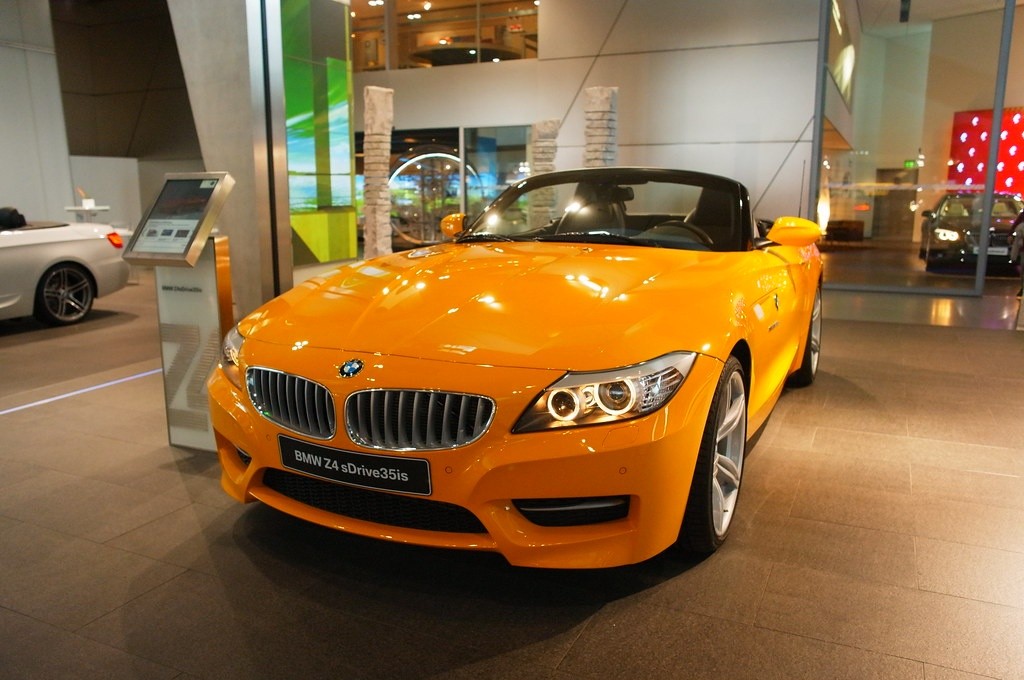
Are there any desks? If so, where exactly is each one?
[64,205,110,223]
[407,42,522,66]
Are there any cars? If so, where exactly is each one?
[919,193,1024,275]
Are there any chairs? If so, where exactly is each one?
[554,183,626,235]
[0,207,27,231]
[683,186,760,248]
[991,203,1009,217]
[948,203,964,216]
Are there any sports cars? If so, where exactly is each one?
[206,166,831,569]
[0,206,130,327]
